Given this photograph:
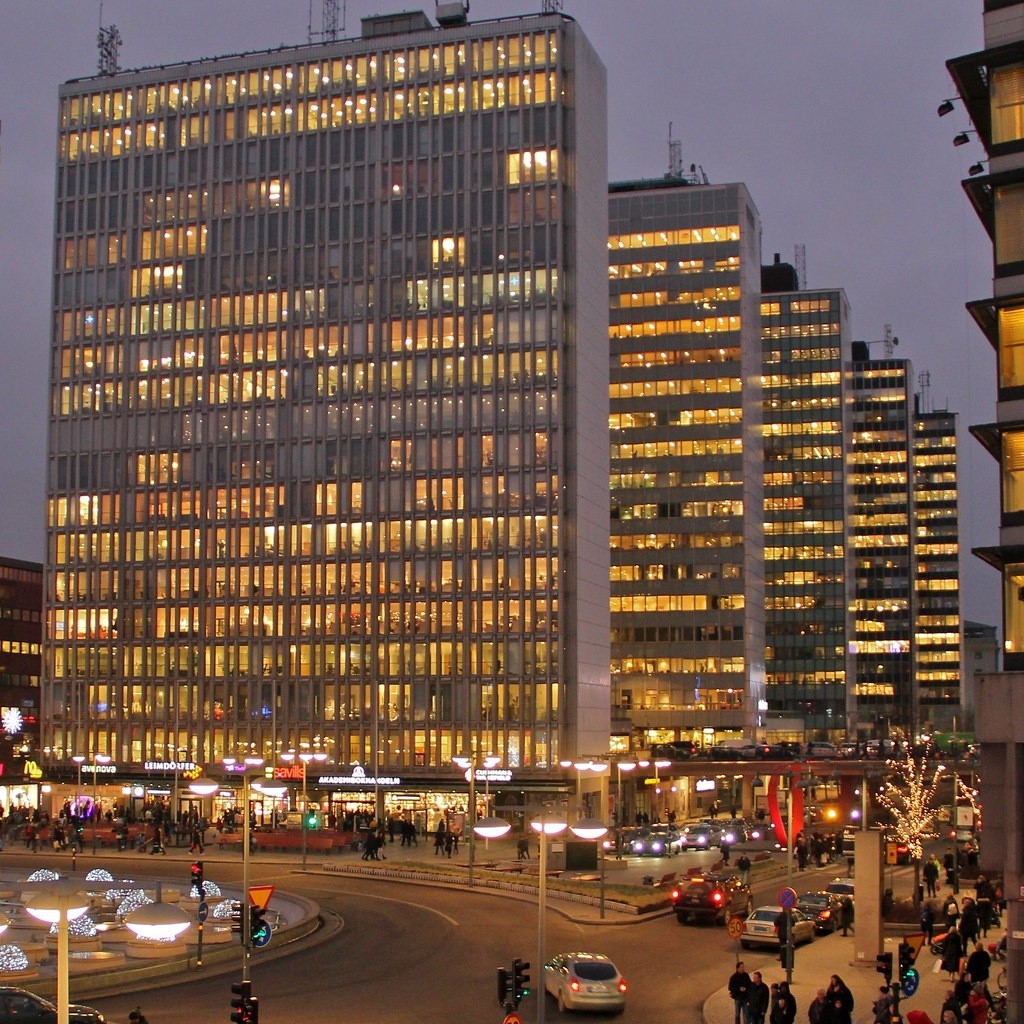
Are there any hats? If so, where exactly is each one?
[772,983,780,989]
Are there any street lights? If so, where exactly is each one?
[280,750,328,873]
[0,879,193,1024]
[471,804,608,1024]
[189,776,288,1024]
[73,751,112,851]
[831,761,888,832]
[751,768,813,985]
[560,755,637,820]
[638,757,672,825]
[222,754,264,860]
[452,750,502,863]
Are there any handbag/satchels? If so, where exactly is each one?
[932,959,943,974]
[957,958,965,977]
[936,880,940,891]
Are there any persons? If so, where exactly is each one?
[611,801,1008,1024]
[0,797,530,860]
[128,1006,144,1024]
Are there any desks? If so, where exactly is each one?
[458,861,607,881]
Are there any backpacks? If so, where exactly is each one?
[920,911,929,924]
[948,903,958,915]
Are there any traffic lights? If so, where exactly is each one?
[191,861,203,886]
[511,958,531,1009]
[309,809,316,829]
[230,904,243,936]
[877,952,893,981]
[230,981,252,1024]
[242,996,258,1024]
[899,943,916,981]
[496,967,512,1005]
[250,905,265,938]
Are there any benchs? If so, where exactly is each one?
[216,826,368,855]
[750,854,764,863]
[731,858,741,867]
[704,862,725,873]
[654,871,677,886]
[23,823,156,848]
[680,866,702,880]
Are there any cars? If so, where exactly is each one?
[602,801,876,955]
[544,952,627,1016]
[648,737,912,761]
[0,986,107,1024]
